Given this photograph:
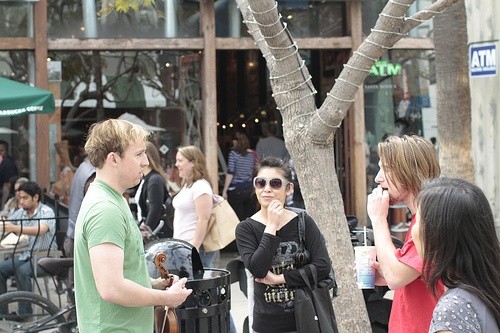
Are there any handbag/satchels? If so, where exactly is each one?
[161,195,175,229]
[253,151,260,174]
[293,263,340,333]
[202,193,241,252]
[297,210,335,288]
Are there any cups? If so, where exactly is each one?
[354,246,377,289]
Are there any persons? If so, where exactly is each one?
[0,140,98,322]
[134,141,169,240]
[235,157,333,333]
[169,147,219,271]
[410,176,500,333]
[354,133,447,333]
[73,120,195,332]
[217,121,299,263]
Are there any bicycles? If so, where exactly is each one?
[0,219,165,333]
[346,214,405,246]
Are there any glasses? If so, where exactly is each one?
[253,177,284,189]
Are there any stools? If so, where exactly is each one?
[27,251,63,322]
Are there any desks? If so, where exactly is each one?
[0,242,28,261]
